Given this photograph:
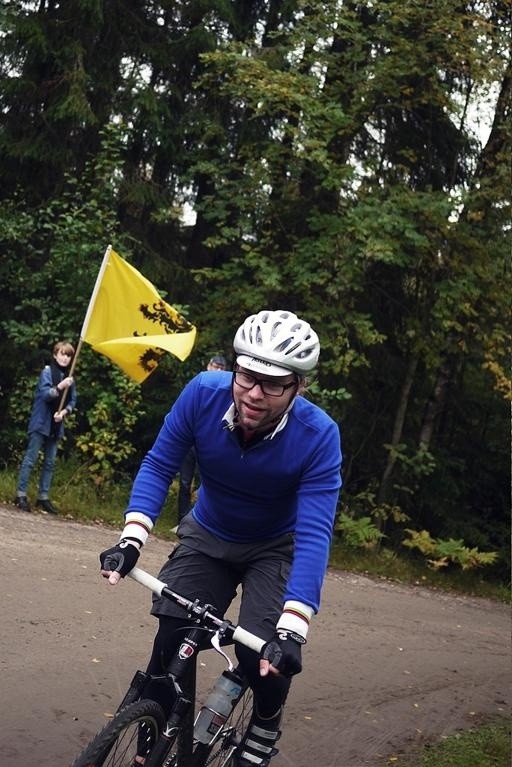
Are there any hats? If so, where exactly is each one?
[211,356,226,368]
[236,355,293,377]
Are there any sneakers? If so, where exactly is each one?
[14,496,58,515]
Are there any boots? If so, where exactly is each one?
[234,703,283,766]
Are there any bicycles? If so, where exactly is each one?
[72,564,268,767]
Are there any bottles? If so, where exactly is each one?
[193,667,245,744]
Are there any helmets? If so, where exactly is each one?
[233,310,320,374]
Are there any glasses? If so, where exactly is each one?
[233,364,298,397]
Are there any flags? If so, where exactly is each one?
[79,247,197,387]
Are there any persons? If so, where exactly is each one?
[14,341,78,515]
[168,354,227,535]
[99,308,343,767]
